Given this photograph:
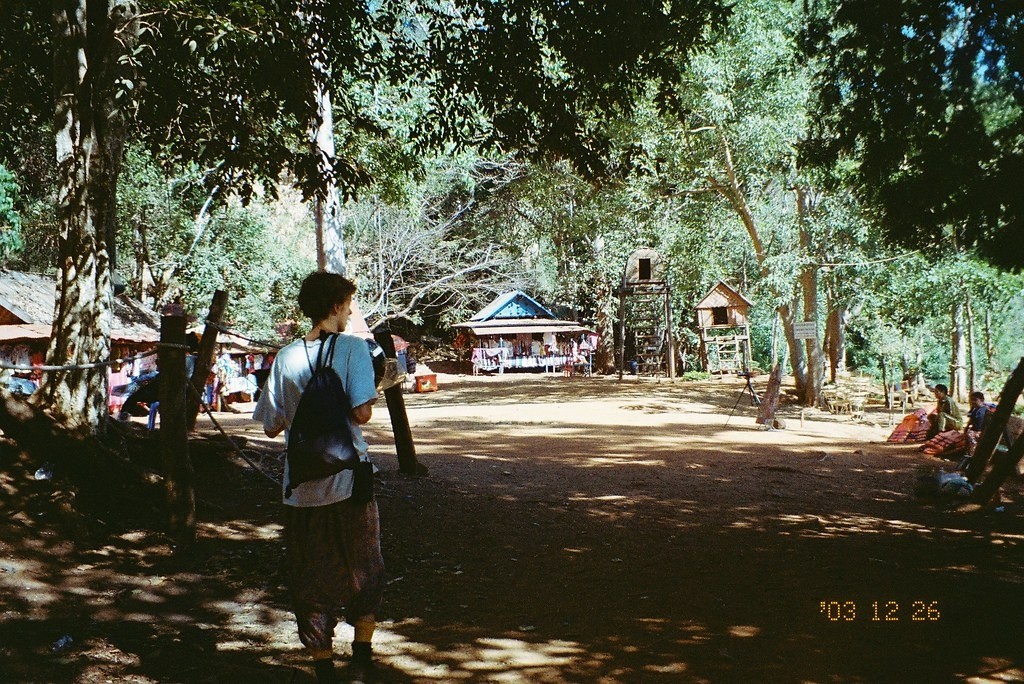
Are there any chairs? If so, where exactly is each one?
[824,390,855,417]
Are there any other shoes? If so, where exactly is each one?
[347,656,376,684]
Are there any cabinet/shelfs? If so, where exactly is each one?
[415,374,438,393]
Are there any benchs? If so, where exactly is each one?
[970,407,1024,477]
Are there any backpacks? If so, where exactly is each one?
[286,333,361,488]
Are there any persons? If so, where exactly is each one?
[252,271,386,684]
[185,331,199,392]
[927,384,989,456]
